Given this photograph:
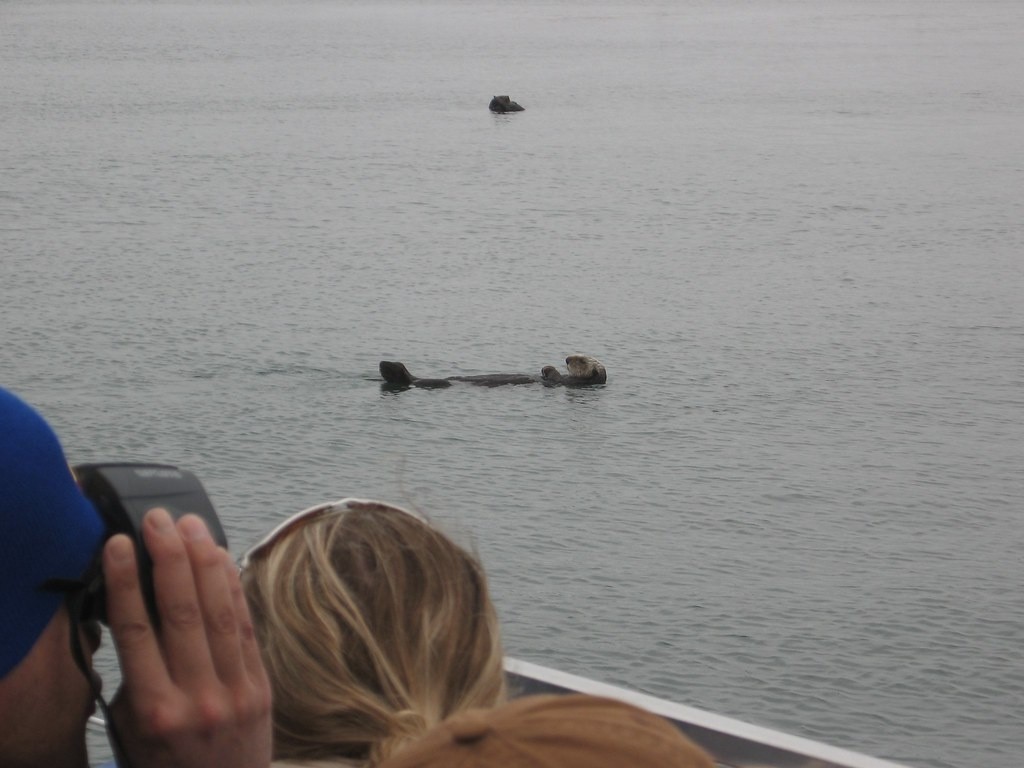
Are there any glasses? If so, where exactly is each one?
[238,492,439,589]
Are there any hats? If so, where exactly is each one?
[0,387,105,680]
[387,690,715,768]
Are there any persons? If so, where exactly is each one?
[0,390,715,768]
